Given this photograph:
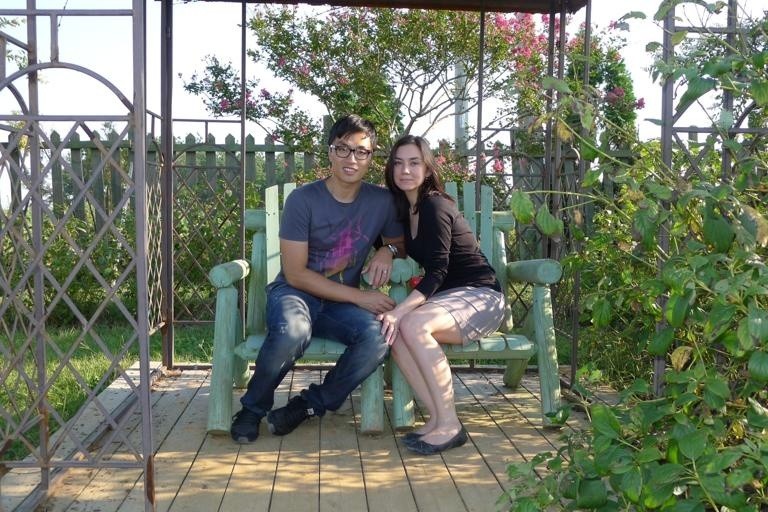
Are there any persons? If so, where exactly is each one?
[374,134,507,455]
[228,112,409,443]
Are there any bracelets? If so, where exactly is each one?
[382,243,400,261]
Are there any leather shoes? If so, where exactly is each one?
[400,424,469,456]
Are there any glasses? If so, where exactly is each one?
[328,144,373,160]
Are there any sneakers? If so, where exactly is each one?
[264,395,312,437]
[230,406,262,445]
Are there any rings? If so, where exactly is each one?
[383,269,388,274]
